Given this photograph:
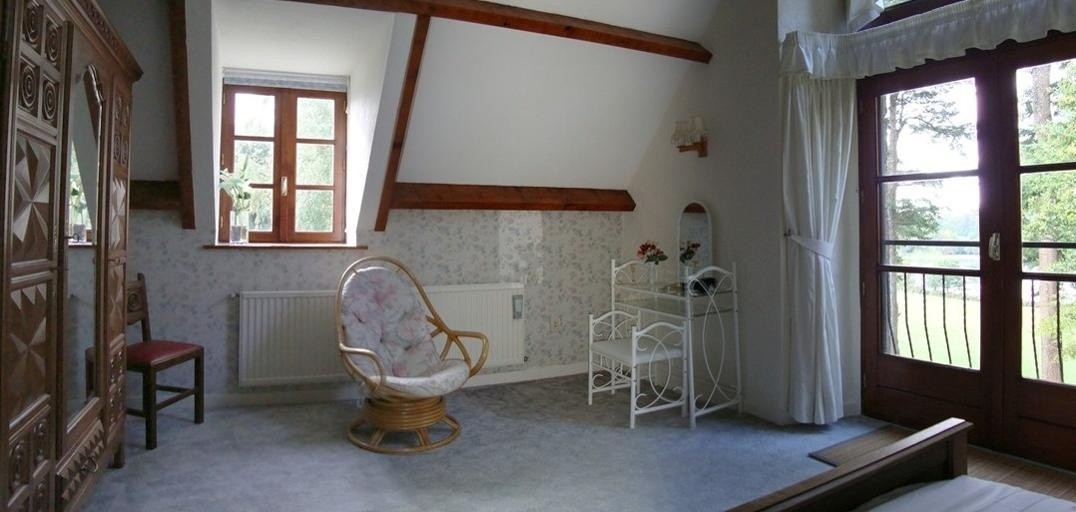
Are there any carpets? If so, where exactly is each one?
[809,426,1075,503]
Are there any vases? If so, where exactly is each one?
[684,266,693,277]
[647,262,660,286]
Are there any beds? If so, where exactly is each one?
[727,417,1076,512]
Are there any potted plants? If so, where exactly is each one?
[218,153,253,246]
[70,165,91,242]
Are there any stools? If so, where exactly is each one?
[588,311,688,429]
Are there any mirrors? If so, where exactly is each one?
[63,64,105,429]
[677,200,713,279]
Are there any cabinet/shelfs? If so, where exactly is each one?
[611,259,743,428]
[0,0,144,512]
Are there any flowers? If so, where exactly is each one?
[680,241,700,266]
[637,241,668,265]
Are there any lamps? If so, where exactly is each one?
[671,114,707,158]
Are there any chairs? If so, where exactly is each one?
[335,255,489,455]
[125,274,204,449]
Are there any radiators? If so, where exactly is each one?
[229,282,527,389]
